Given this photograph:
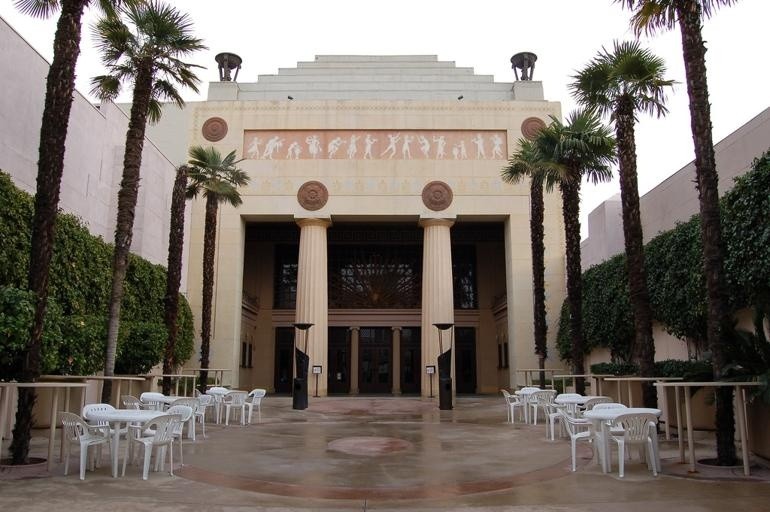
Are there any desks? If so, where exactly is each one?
[0,368,232,480]
[516,366,768,478]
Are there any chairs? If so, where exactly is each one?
[220,389,266,426]
[500,389,528,424]
